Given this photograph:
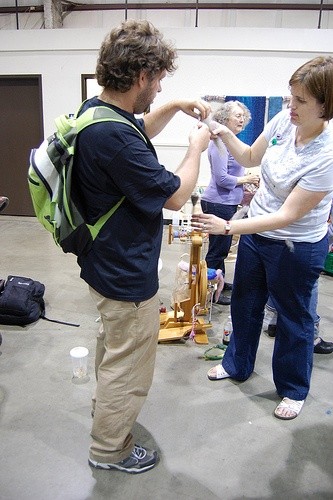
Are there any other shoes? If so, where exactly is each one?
[263,324,276,338]
[210,291,231,305]
[314,337,333,354]
[223,282,233,290]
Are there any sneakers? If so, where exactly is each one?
[88,443,157,475]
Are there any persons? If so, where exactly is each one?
[191,56,333,420]
[200,101,252,306]
[265,277,333,354]
[66,22,213,473]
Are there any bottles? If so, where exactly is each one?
[223,316,233,346]
[159,302,167,313]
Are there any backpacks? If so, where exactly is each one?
[28,95,148,256]
[0,275,46,327]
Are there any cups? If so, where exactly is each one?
[70,346,89,378]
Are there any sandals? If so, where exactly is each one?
[207,364,232,381]
[274,397,305,420]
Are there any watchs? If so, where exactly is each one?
[224,222,230,236]
